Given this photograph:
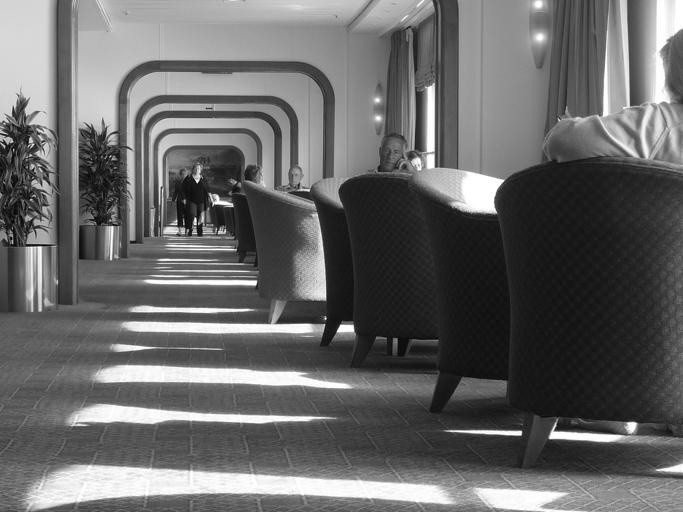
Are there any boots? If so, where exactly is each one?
[176,232,203,237]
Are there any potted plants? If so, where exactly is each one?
[78,113,134,262]
[0,79,61,313]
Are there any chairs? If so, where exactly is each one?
[241,180,327,324]
[408,167,576,430]
[209,181,312,267]
[309,174,416,359]
[494,156,681,469]
[337,167,439,370]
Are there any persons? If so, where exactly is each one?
[541,31,683,160]
[224,165,308,192]
[369,133,421,173]
[171,163,215,236]
